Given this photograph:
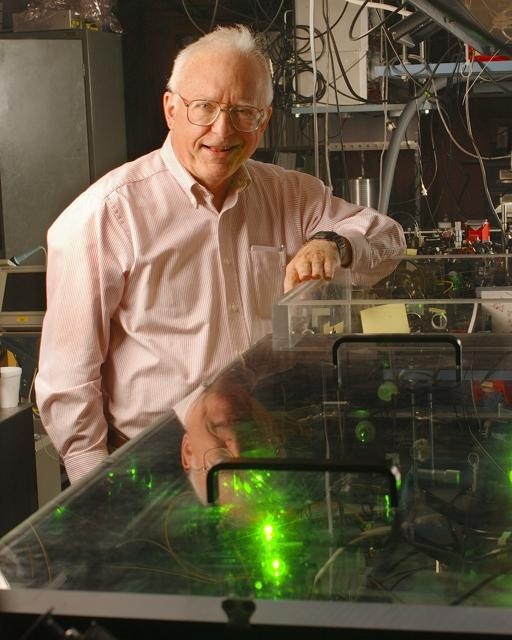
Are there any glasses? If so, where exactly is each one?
[190,447,233,474]
[178,95,273,134]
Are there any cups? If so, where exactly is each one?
[1,366,23,409]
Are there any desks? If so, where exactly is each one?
[0,405,36,535]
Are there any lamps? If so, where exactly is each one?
[8,245,48,267]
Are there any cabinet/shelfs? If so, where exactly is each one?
[0,31,129,267]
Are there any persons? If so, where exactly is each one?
[35,24,409,560]
[172,337,375,529]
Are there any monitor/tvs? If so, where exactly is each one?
[0,266,47,332]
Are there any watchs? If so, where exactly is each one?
[309,229,346,261]
